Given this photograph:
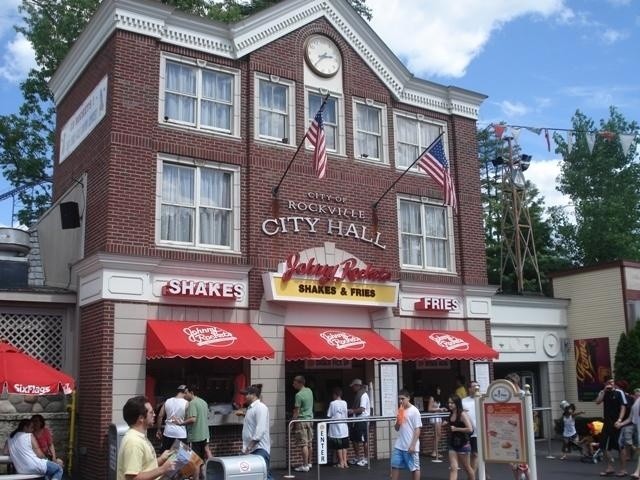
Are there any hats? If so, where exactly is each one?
[240,386,259,396]
[349,379,363,387]
[177,384,187,391]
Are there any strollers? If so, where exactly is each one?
[570,417,614,464]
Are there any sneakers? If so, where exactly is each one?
[294,463,313,472]
[348,458,369,467]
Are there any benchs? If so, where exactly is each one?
[0,474,48,480]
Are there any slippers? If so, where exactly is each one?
[560,456,640,479]
[332,462,349,469]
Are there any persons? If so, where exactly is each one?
[347,378,370,467]
[428,378,480,480]
[291,375,313,472]
[3,418,63,480]
[31,414,64,467]
[155,384,212,480]
[326,387,349,469]
[116,396,176,480]
[505,372,530,480]
[241,386,273,480]
[560,376,640,480]
[391,389,421,480]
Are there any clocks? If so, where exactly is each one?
[302,32,343,79]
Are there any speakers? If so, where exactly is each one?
[59,201,80,229]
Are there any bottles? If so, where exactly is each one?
[397,404,404,425]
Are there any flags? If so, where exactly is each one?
[417,137,458,215]
[306,106,328,179]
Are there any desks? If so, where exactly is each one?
[0,454,19,473]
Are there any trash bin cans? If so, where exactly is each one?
[205,454,267,480]
[108,423,133,480]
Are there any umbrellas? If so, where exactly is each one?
[1,340,76,396]
